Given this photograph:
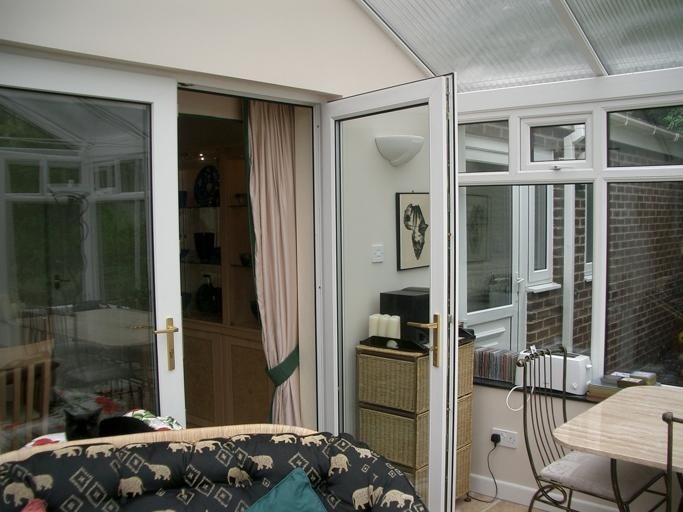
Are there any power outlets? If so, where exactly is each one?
[489,426,519,451]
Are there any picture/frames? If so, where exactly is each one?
[461,191,491,266]
[391,188,432,274]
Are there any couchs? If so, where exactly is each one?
[1,412,430,511]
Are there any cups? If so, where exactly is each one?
[368,313,403,341]
[178,190,187,208]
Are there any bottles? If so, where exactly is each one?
[196,274,215,315]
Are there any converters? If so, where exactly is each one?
[491,434,500,442]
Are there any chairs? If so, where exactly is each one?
[657,411,682,512]
[510,344,670,511]
[9,296,158,418]
[0,337,59,457]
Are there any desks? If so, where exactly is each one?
[549,383,682,512]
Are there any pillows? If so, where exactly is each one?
[242,462,328,511]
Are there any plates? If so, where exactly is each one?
[193,165,219,207]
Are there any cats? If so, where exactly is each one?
[63,408,156,441]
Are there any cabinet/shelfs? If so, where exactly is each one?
[168,152,280,431]
[354,330,476,507]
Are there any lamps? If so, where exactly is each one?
[373,133,425,170]
